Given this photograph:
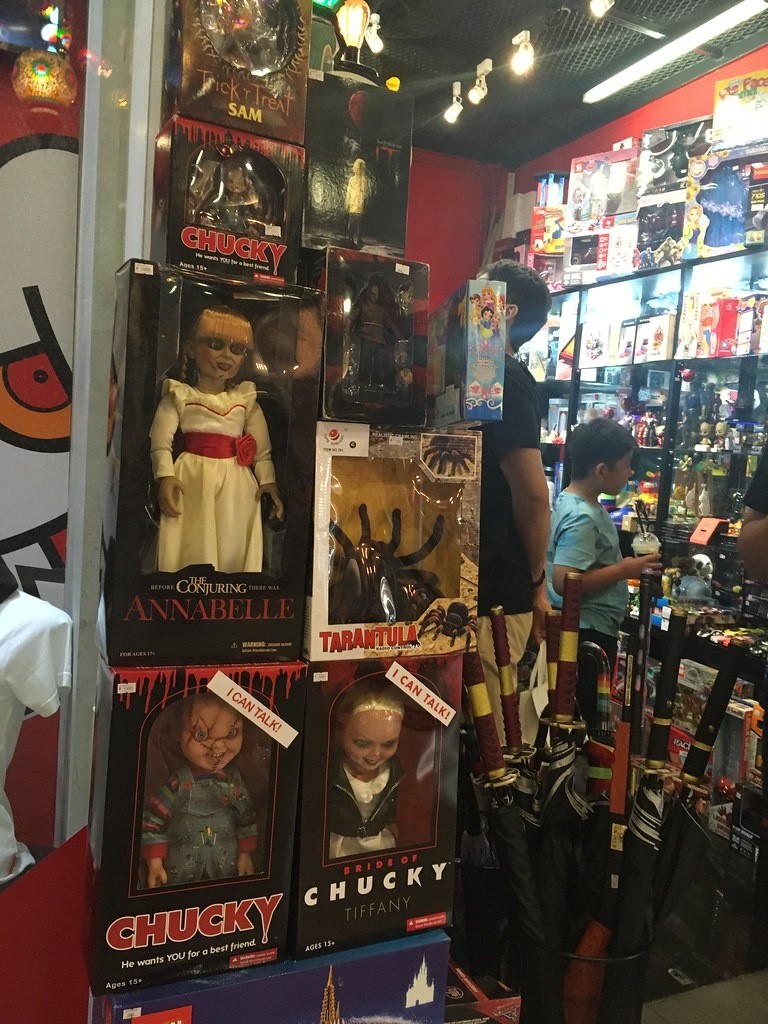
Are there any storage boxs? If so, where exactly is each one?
[547,392,619,439]
[610,631,765,864]
[579,293,768,368]
[87,1,521,1024]
[500,67,768,290]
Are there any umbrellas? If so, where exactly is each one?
[455,605,746,1024]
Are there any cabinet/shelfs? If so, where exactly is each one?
[522,244,768,694]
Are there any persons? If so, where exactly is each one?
[464,259,552,753]
[149,305,285,573]
[574,377,731,453]
[141,692,259,888]
[344,284,405,390]
[736,442,768,584]
[544,419,662,723]
[329,680,405,859]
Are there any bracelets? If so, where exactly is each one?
[532,569,545,587]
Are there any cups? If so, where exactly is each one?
[630,543,662,558]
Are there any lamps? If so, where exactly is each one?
[444,82,464,123]
[468,58,493,104]
[511,30,534,74]
[13,47,78,118]
